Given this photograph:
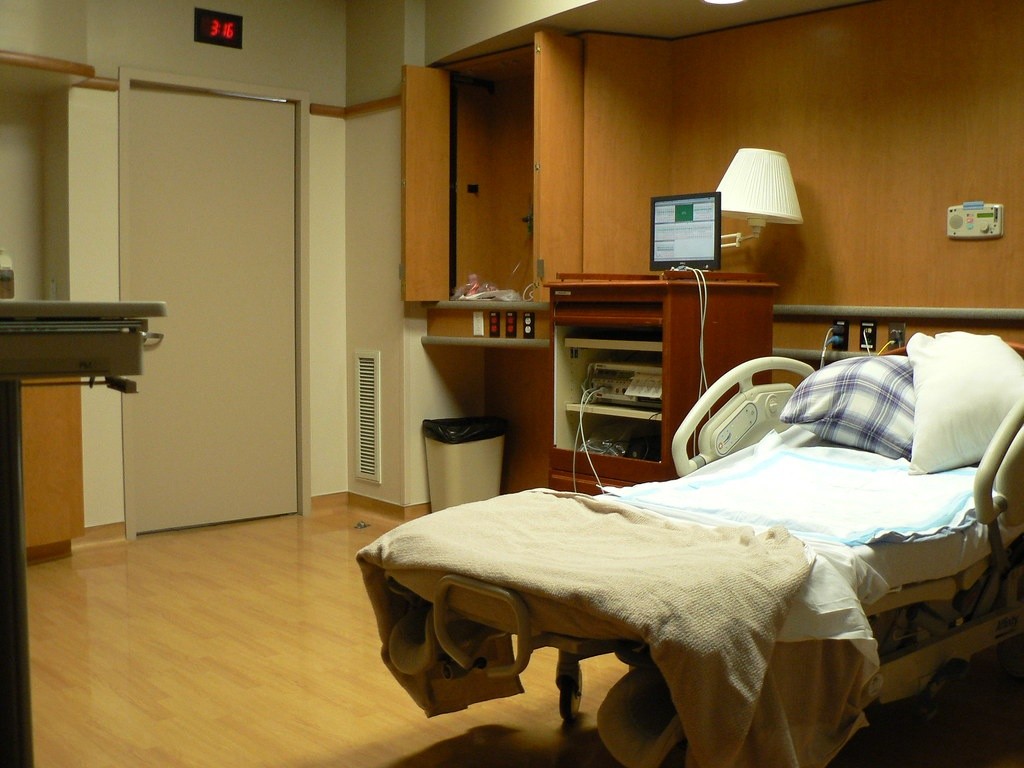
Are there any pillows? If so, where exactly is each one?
[780,355,914,462]
[906,331,1024,475]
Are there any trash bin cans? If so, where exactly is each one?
[422,413,507,514]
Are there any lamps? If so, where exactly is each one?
[716,148,803,247]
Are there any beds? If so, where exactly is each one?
[356,357,1024,768]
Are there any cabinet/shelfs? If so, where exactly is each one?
[21,376,87,567]
[542,279,780,496]
[399,27,673,302]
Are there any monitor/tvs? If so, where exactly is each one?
[650,192,722,271]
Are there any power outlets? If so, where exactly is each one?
[888,322,906,351]
[831,319,850,351]
[472,311,484,336]
[859,321,877,351]
